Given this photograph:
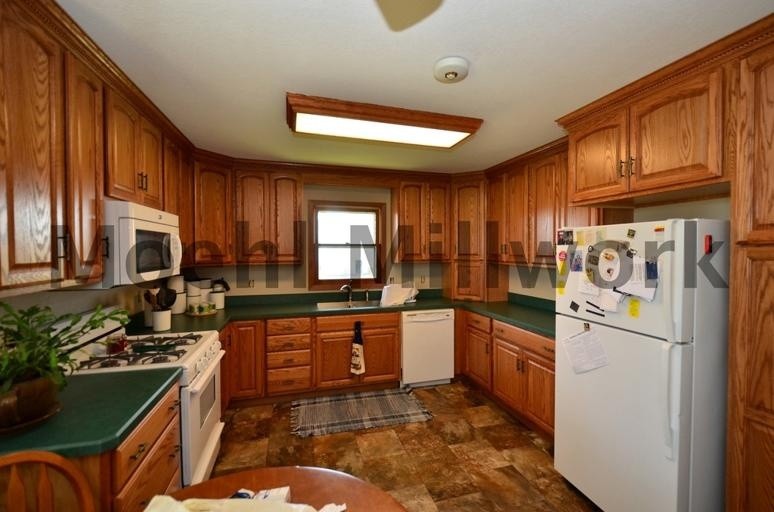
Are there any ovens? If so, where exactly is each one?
[180,349,225,490]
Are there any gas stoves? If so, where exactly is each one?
[57,327,222,387]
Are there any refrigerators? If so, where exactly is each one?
[551,216,730,511]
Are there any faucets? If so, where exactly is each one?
[339,279,354,303]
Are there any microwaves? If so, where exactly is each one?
[55,199,185,292]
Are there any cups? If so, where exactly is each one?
[152,308,172,332]
[167,276,226,315]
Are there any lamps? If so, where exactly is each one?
[281,92,483,153]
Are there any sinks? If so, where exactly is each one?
[316,300,381,310]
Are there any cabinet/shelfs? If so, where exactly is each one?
[554,36,730,212]
[389,170,425,265]
[218,320,232,419]
[423,170,452,265]
[442,167,510,304]
[552,135,635,270]
[489,315,555,454]
[311,308,403,399]
[229,316,269,408]
[2,0,308,302]
[524,138,559,272]
[721,8,774,512]
[266,314,317,406]
[500,152,531,271]
[482,161,510,268]
[455,308,494,402]
[0,379,185,512]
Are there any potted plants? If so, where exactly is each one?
[2,302,131,434]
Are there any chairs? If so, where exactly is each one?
[1,442,97,511]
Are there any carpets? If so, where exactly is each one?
[289,380,435,444]
